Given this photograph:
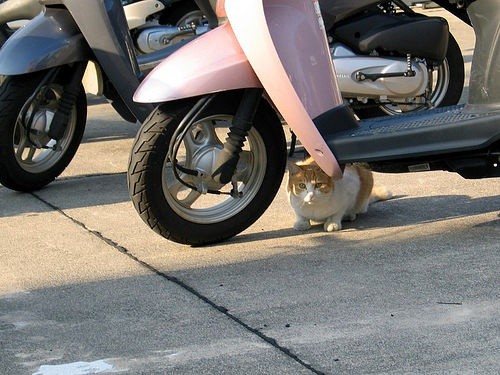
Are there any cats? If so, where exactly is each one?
[287,156,374,232]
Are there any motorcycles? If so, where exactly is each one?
[126,0,500,247]
[0,0,465,192]
